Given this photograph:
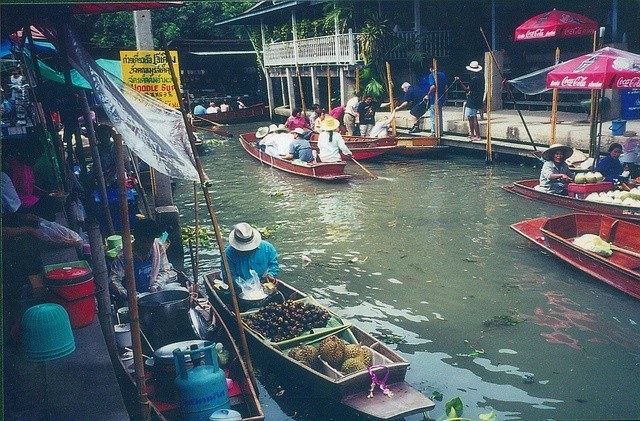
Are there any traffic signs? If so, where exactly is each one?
[119,50,184,110]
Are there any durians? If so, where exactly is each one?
[362,348,373,367]
[344,341,362,360]
[288,342,321,373]
[342,358,367,373]
[320,335,345,371]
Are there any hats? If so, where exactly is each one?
[256,127,269,139]
[321,115,340,131]
[276,123,289,132]
[542,143,574,162]
[466,60,483,73]
[291,127,306,136]
[269,124,279,134]
[229,223,262,252]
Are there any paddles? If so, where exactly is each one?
[349,155,378,182]
[193,126,233,139]
[193,115,230,127]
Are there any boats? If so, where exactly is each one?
[111,265,265,420]
[203,269,435,421]
[509,212,640,298]
[498,178,640,220]
[187,102,269,125]
[384,137,450,155]
[238,132,352,182]
[306,132,399,161]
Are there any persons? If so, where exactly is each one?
[92,123,131,188]
[329,105,346,120]
[221,222,279,307]
[110,218,170,300]
[369,109,395,138]
[357,93,391,136]
[539,143,574,194]
[286,107,312,138]
[424,62,449,136]
[317,116,355,162]
[501,31,530,68]
[454,60,485,140]
[253,124,294,158]
[286,126,315,163]
[315,110,325,132]
[193,98,247,114]
[7,66,30,104]
[395,80,431,132]
[343,91,363,135]
[310,103,320,132]
[596,142,629,184]
[1,135,44,241]
